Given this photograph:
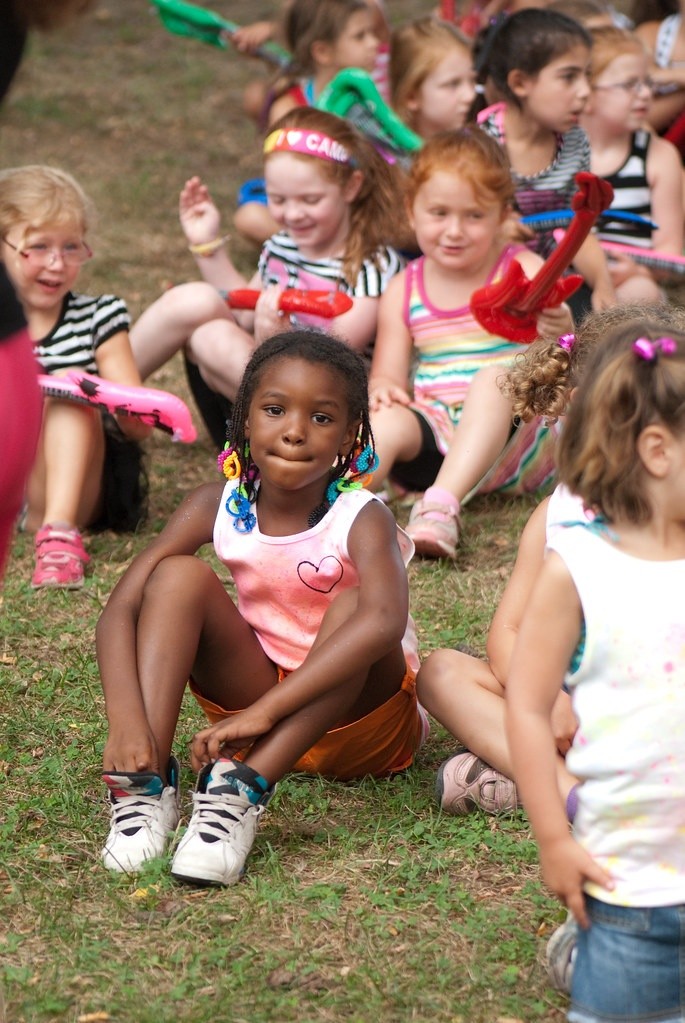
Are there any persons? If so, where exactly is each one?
[130,106,404,449]
[416,304,685,814]
[333,127,583,557]
[234,1,685,322]
[0,166,151,590]
[504,322,685,1023]
[96,330,430,885]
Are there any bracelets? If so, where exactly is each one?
[187,236,234,254]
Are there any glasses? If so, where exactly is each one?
[592,75,657,95]
[1,233,93,271]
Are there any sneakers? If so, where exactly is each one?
[29,520,91,589]
[434,752,523,817]
[101,756,180,870]
[169,758,270,887]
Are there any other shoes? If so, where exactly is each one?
[405,497,463,560]
